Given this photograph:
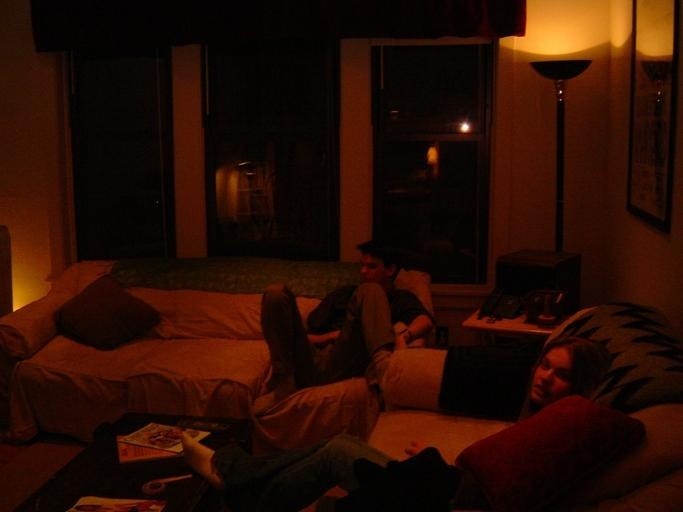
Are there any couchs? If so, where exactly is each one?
[334,302,682,512]
[1,260,437,449]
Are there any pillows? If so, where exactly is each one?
[452,391,646,511]
[53,275,162,351]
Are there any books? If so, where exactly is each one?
[119,422,211,464]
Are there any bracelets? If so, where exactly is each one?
[404,328,413,338]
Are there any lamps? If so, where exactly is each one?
[528,59,593,252]
[641,60,671,202]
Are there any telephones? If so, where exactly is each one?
[480,288,524,319]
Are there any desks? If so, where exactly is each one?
[462,307,554,418]
[10,412,254,512]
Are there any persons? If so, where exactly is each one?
[180,337,610,512]
[250,236,453,409]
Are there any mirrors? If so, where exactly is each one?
[624,1,682,233]
